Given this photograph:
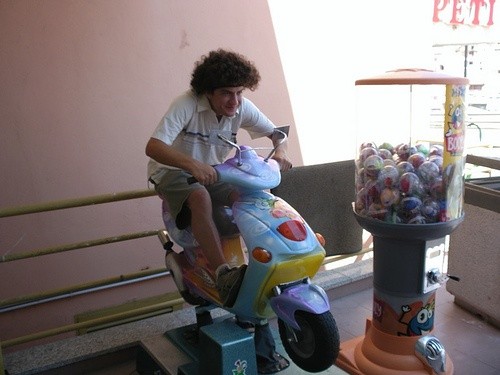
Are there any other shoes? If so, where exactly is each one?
[273,351,290,371]
[217,263,248,307]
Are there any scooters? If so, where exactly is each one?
[151,125,341,373]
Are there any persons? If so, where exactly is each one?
[145,50,292,309]
